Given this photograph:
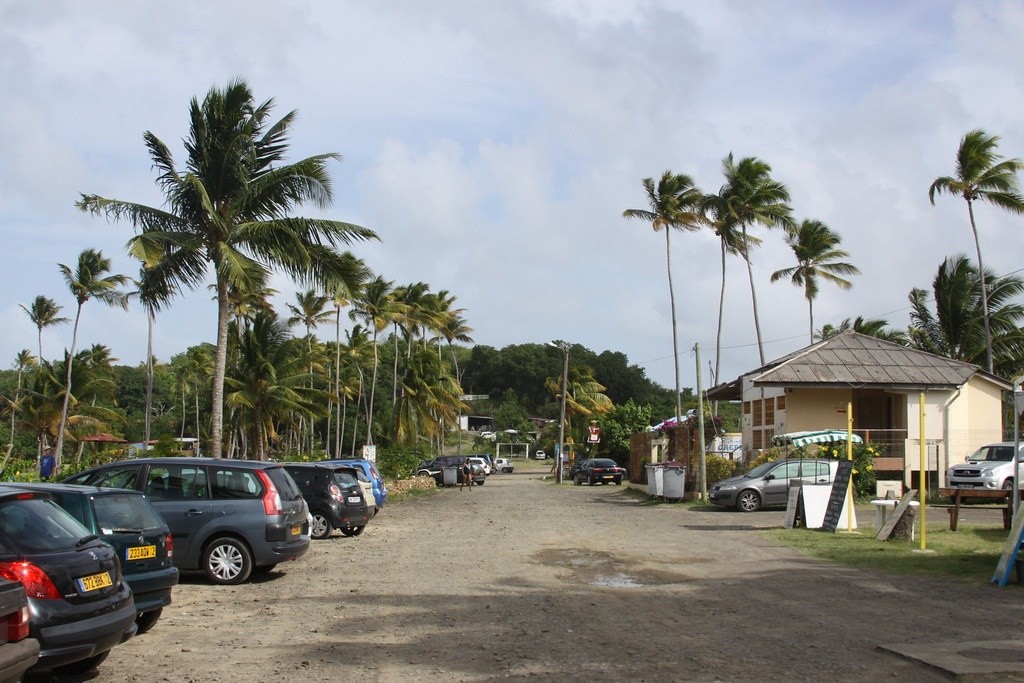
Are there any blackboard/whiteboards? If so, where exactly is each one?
[822,459,853,533]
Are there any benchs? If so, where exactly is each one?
[930,489,1024,532]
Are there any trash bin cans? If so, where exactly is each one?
[645,461,686,503]
[441,465,458,487]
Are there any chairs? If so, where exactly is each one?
[149,476,165,498]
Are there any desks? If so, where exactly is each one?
[870,499,920,541]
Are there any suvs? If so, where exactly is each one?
[466,454,495,474]
[417,455,470,484]
[0,485,137,683]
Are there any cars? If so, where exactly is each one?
[574,459,623,486]
[6,485,180,633]
[569,460,627,482]
[263,458,385,540]
[440,464,486,485]
[468,458,491,475]
[0,576,39,681]
[494,458,513,474]
[536,450,546,460]
[945,443,1024,502]
[480,431,497,439]
[709,458,840,512]
[56,459,315,585]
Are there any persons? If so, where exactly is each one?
[40,446,56,479]
[460,458,472,491]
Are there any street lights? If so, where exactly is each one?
[548,341,569,484]
